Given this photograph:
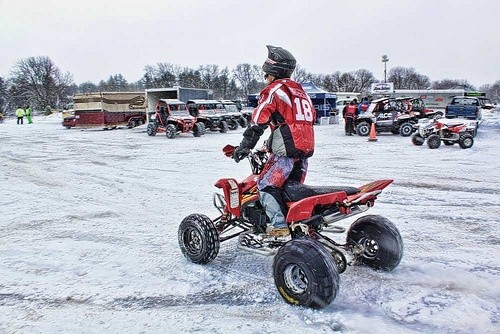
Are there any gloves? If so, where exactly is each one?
[233,144,251,163]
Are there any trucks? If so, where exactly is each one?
[370,83,395,97]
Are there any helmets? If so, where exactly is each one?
[262,45,296,78]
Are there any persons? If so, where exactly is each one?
[15,104,33,124]
[342,98,373,137]
[233,46,317,242]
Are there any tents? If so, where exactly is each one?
[247,81,337,117]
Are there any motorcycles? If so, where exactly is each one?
[411,116,479,149]
[176,140,405,310]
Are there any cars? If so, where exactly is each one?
[217,98,248,129]
[146,98,202,139]
[356,96,443,137]
[186,99,228,135]
[479,97,497,109]
[208,100,239,130]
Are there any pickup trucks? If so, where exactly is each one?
[444,96,483,121]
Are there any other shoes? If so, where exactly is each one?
[257,226,292,243]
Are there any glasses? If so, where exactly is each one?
[264,73,269,78]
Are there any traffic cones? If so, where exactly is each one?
[367,123,378,141]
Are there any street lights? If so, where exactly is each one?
[381,54,389,83]
